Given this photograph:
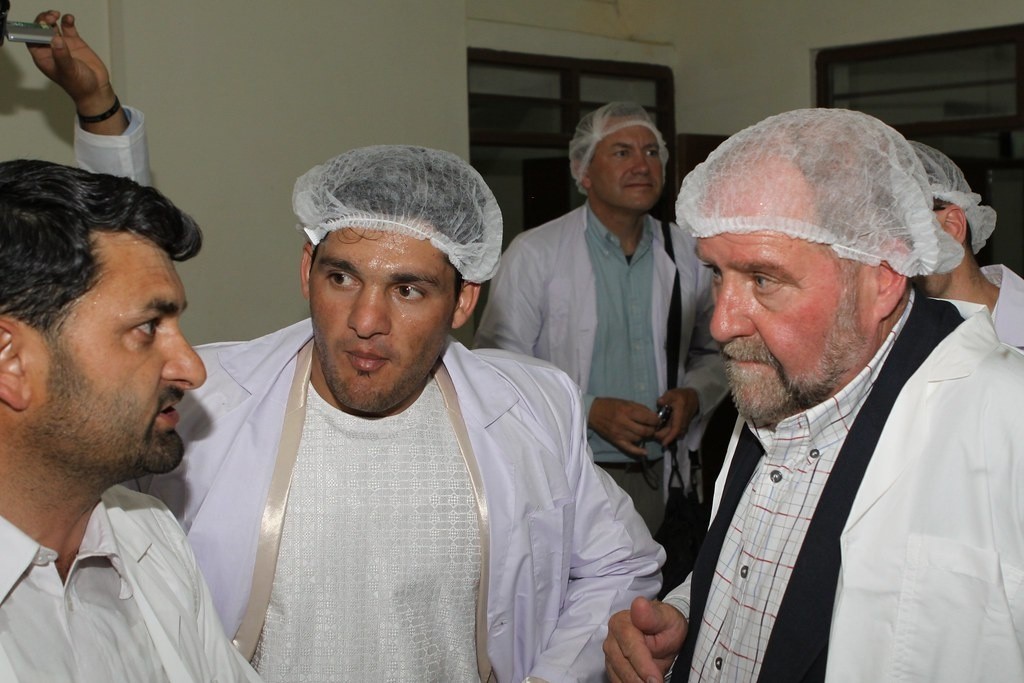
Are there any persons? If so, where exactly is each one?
[0,0,1024,683]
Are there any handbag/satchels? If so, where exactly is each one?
[652,485,703,602]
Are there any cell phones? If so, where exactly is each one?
[655,403,669,431]
[5,21,55,44]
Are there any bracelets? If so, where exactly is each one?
[75,93,120,123]
